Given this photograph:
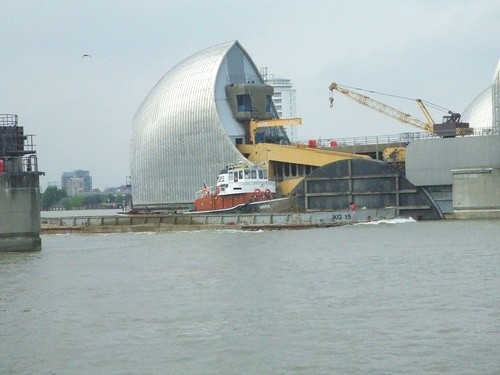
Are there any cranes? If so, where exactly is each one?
[328,81,473,163]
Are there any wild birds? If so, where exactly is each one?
[82,54,90,59]
